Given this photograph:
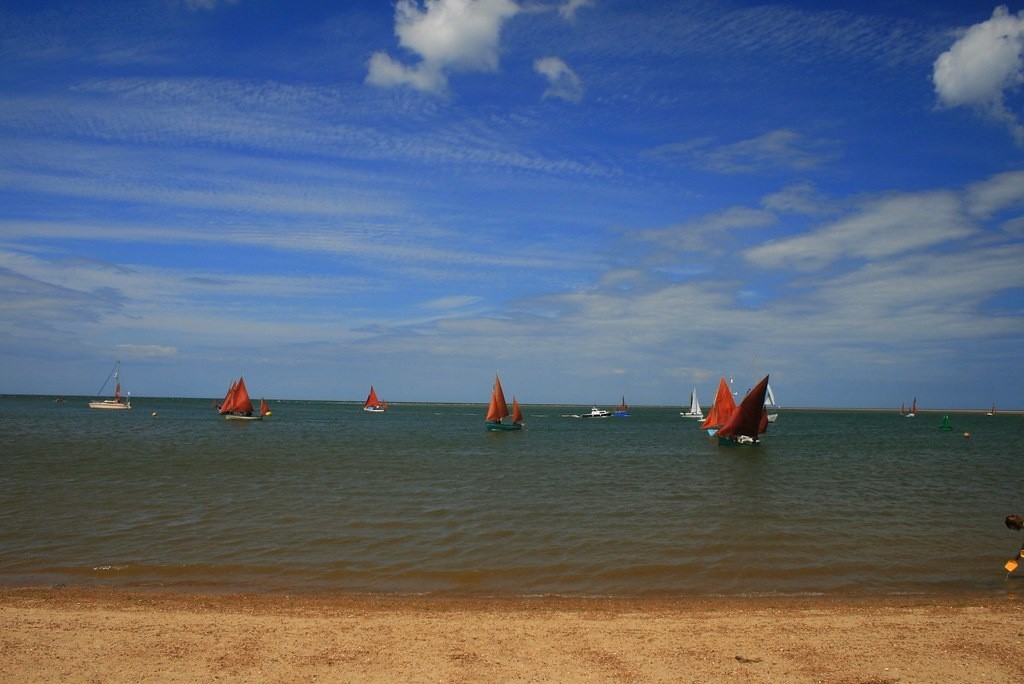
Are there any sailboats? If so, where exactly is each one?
[698,374,779,444]
[900,398,917,419]
[620,396,629,411]
[88,360,133,410]
[364,385,388,413]
[986,405,996,416]
[485,374,523,431]
[679,387,704,418]
[216,376,270,420]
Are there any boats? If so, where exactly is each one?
[582,406,612,418]
[939,415,953,431]
